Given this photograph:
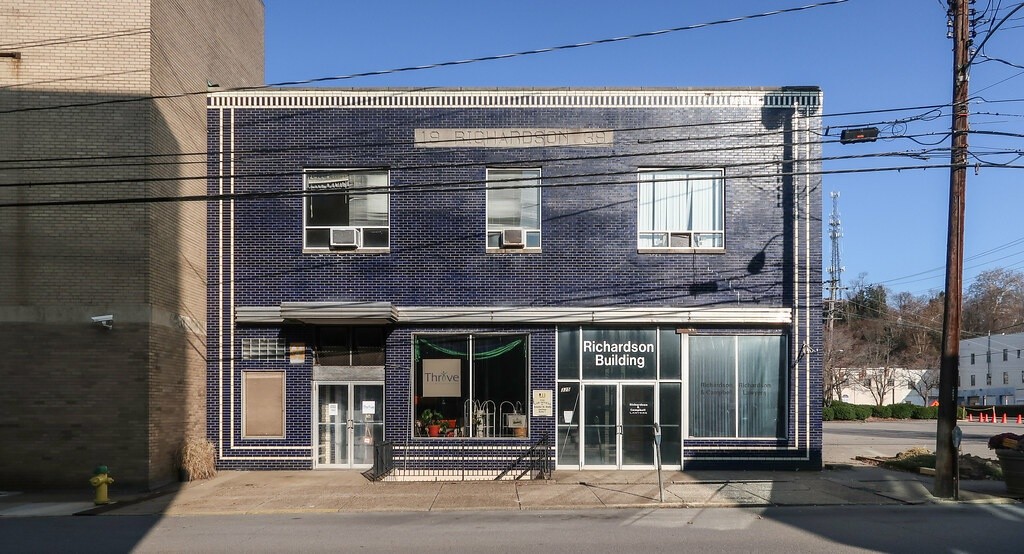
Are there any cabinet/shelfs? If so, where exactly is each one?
[464,400,495,437]
[499,401,523,437]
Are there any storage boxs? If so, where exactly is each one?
[505,414,527,428]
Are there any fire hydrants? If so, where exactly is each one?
[91,465,114,503]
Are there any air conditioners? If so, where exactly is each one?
[330,228,360,247]
[502,229,525,246]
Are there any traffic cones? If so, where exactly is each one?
[992,414,997,423]
[968,413,973,422]
[1001,413,1007,423]
[979,413,989,423]
[1016,415,1022,424]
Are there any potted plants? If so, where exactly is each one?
[422,409,444,437]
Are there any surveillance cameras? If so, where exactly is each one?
[91,315,114,323]
